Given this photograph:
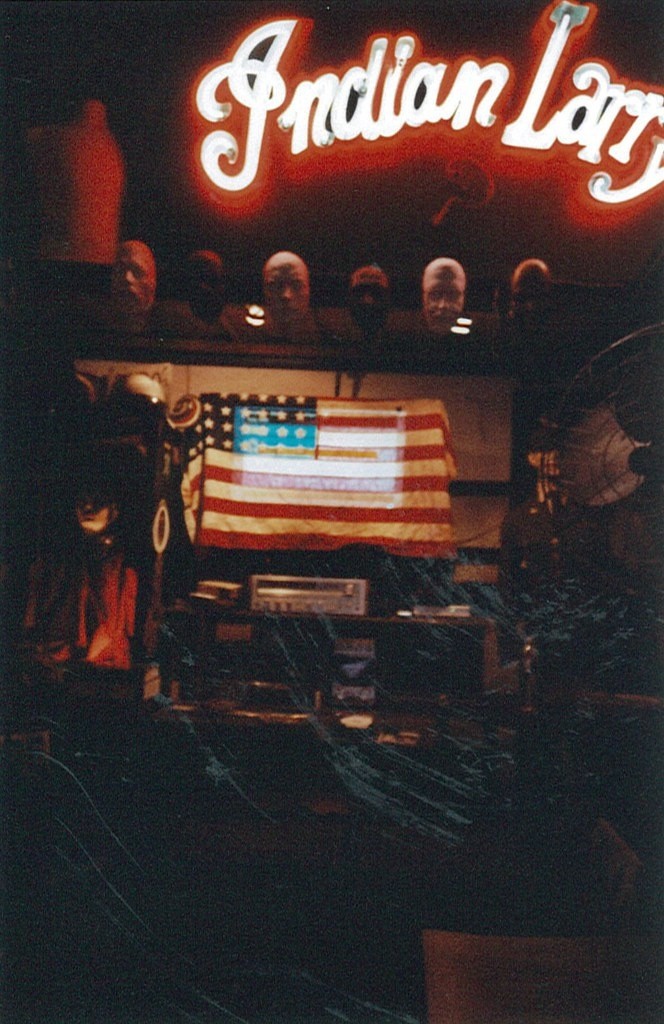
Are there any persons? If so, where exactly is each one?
[18,239,642,671]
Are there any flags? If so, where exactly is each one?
[180,392,458,560]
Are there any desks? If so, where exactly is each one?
[198,608,497,715]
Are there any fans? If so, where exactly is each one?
[538,322,664,600]
